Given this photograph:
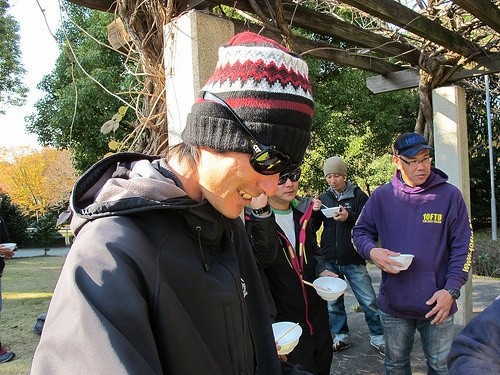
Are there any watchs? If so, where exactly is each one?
[447,288,460,300]
[252,203,270,215]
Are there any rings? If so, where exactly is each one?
[384,269,386,271]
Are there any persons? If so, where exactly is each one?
[352,132,474,375]
[30,33,314,375]
[446,296,499,375]
[243,156,390,375]
[0,197,15,363]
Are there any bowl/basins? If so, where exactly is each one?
[321,207,341,218]
[312,276,347,300]
[388,254,414,270]
[1,243,17,252]
[272,321,302,355]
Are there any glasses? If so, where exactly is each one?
[278,169,301,184]
[204,92,300,178]
[398,156,432,168]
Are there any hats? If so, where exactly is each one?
[324,157,346,178]
[181,33,314,164]
[395,132,434,157]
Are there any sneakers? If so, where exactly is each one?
[370,340,387,356]
[331,338,351,354]
[0,347,15,363]
